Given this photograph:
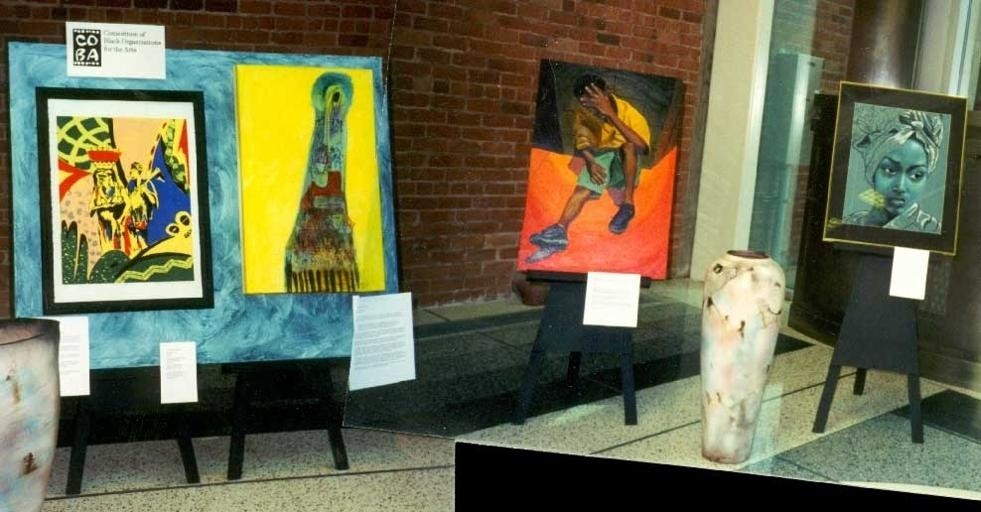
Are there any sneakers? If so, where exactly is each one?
[529,223,568,247]
[607,204,634,233]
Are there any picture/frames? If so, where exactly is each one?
[821,79,970,256]
[31,84,216,317]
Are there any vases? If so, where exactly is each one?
[0,319,62,512]
[700,250,786,465]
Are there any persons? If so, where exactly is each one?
[842,104,948,237]
[530,73,652,247]
